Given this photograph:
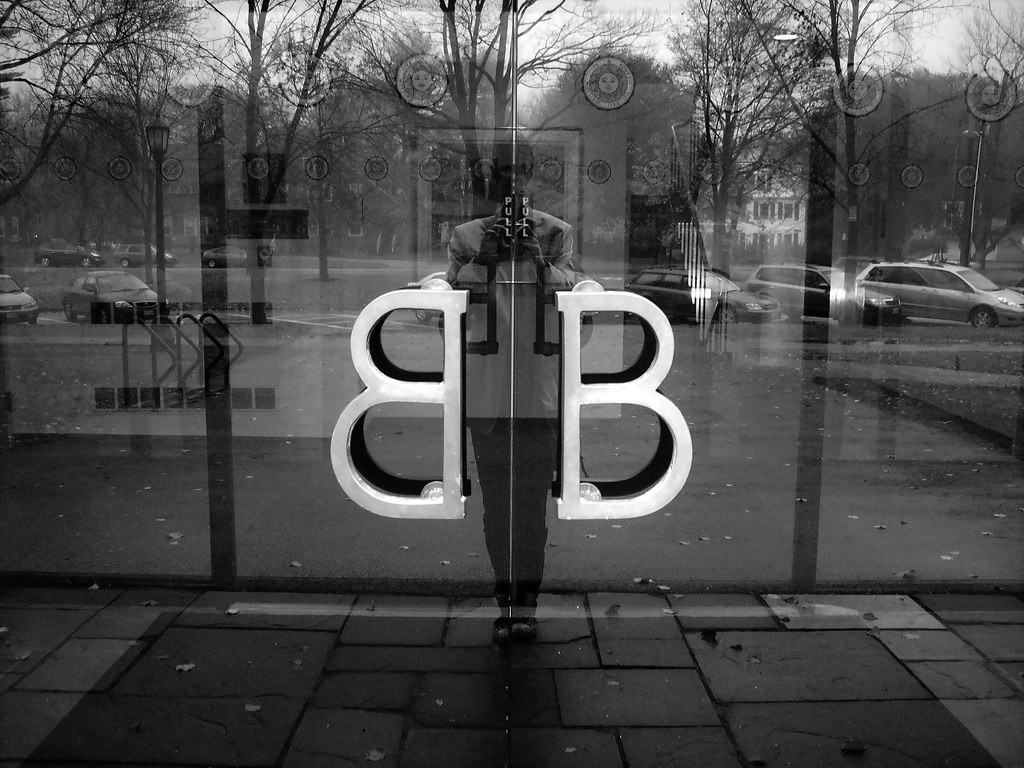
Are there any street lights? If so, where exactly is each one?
[143,112,177,352]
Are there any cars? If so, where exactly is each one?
[0,274,41,326]
[34,239,105,269]
[202,245,272,268]
[824,256,903,289]
[112,244,178,269]
[58,271,170,326]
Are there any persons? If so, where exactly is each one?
[445,127,589,638]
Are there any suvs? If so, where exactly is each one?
[625,266,784,324]
[851,259,1024,329]
[746,261,902,327]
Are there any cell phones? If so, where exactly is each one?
[503,194,533,240]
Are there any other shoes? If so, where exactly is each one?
[492,615,510,644]
[511,616,537,642]
